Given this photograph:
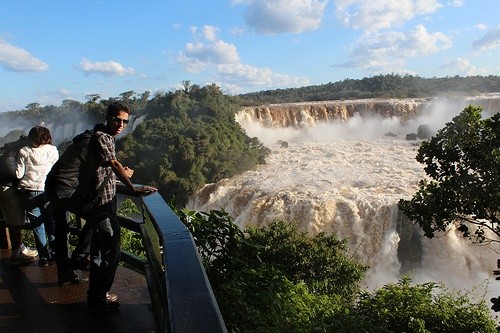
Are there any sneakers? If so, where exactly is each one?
[21,245,38,257]
[10,253,35,265]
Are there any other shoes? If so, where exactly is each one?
[69,260,91,272]
[87,292,118,305]
[58,276,88,286]
[39,259,51,269]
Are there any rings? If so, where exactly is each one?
[148,187,150,190]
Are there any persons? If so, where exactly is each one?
[88,101,159,303]
[0,134,39,264]
[46,122,135,285]
[6,125,59,266]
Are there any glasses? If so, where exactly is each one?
[110,116,129,124]
